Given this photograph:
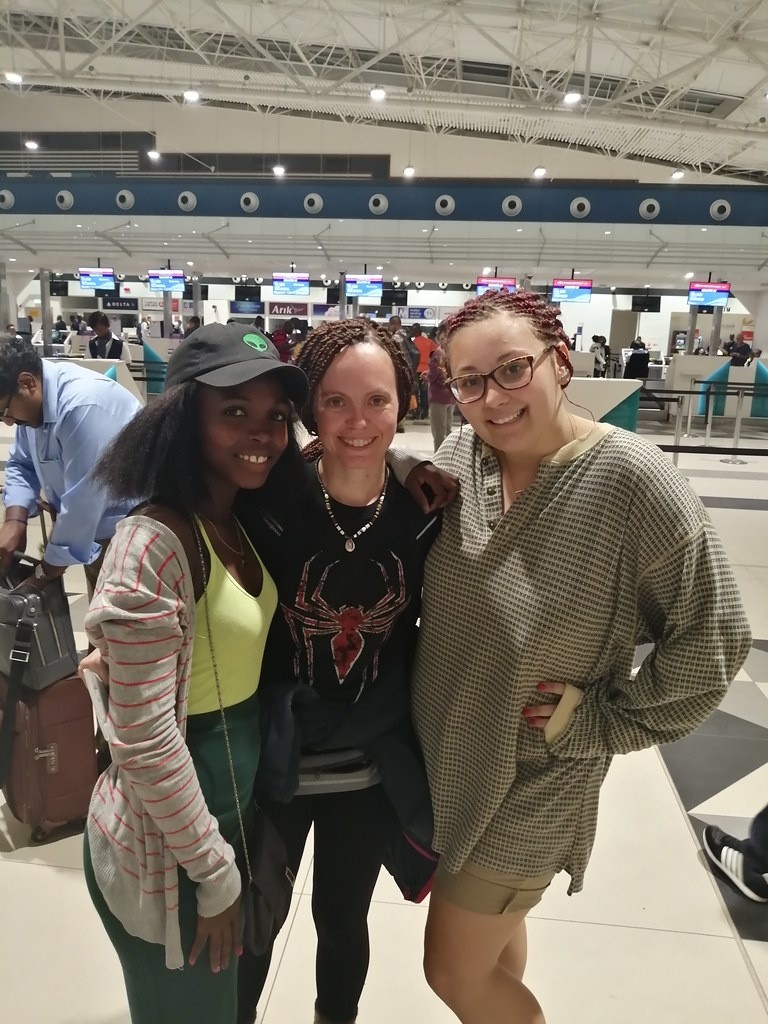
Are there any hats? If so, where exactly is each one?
[164,321,310,407]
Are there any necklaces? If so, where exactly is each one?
[197,509,247,568]
[316,454,389,552]
[505,462,526,495]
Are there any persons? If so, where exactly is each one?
[229,314,457,453]
[136,316,203,341]
[0,334,146,656]
[77,314,443,1024]
[590,335,647,378]
[694,334,761,367]
[8,313,132,370]
[703,806,768,902]
[409,288,752,1024]
[84,321,315,1024]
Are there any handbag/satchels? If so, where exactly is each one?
[0,548,79,691]
[235,808,296,958]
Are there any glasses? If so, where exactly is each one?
[0,369,30,422]
[444,341,558,405]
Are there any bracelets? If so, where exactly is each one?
[3,518,28,526]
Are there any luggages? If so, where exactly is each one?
[0,673,101,843]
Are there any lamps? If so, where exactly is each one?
[184,0,199,99]
[146,132,160,159]
[5,37,23,84]
[534,167,547,177]
[370,13,386,100]
[672,122,684,179]
[403,123,416,177]
[566,0,581,104]
[272,89,286,176]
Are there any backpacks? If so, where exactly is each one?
[394,331,420,374]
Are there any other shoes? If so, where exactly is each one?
[396,428,405,433]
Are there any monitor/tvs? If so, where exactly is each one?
[687,281,731,314]
[148,269,186,291]
[632,296,661,312]
[79,267,115,290]
[476,277,516,298]
[272,272,310,295]
[50,281,68,296]
[183,285,208,300]
[551,279,592,303]
[381,290,408,306]
[345,274,383,297]
[235,286,261,302]
[95,283,120,298]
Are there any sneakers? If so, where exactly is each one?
[703,826,768,904]
